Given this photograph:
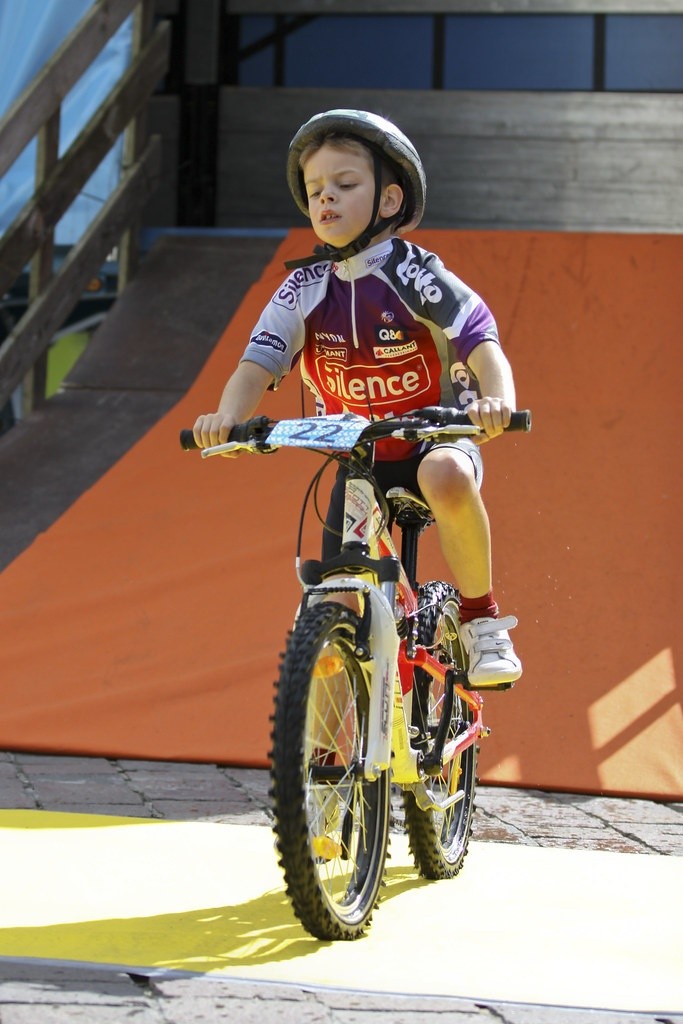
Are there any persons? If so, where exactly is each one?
[194,109,523,863]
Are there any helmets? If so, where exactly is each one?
[287,109,427,235]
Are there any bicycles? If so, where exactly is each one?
[179,405,531,942]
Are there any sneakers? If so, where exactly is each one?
[300,782,340,846]
[459,615,522,687]
